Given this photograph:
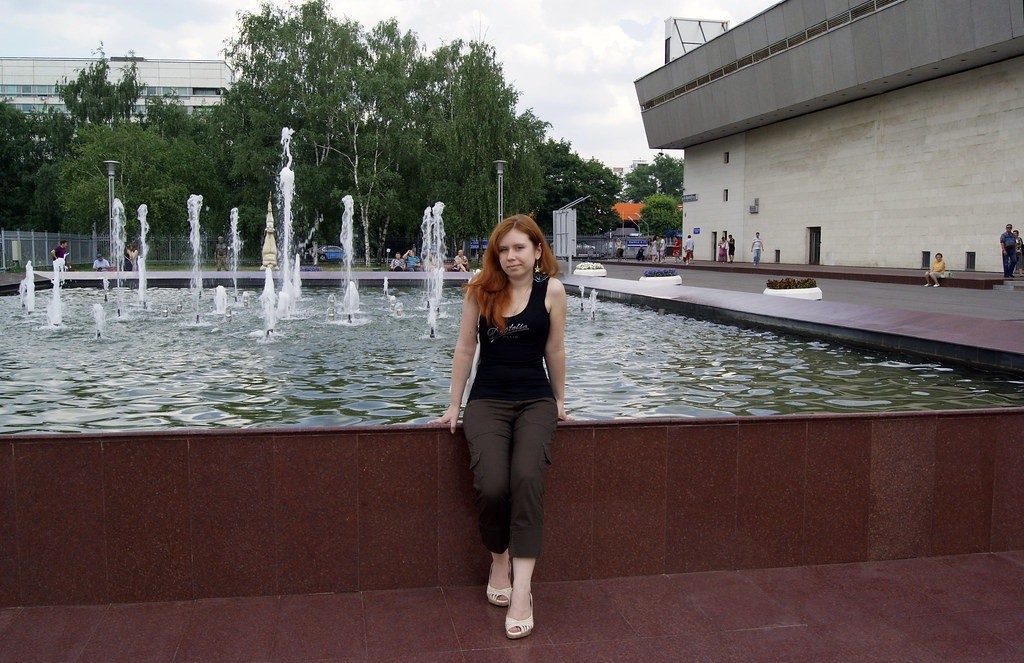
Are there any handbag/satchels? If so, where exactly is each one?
[941,271,952,278]
[721,241,728,250]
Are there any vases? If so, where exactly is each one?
[573,268,609,277]
[763,285,822,299]
[640,275,682,287]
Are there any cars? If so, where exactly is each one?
[577,240,595,254]
[303,245,344,263]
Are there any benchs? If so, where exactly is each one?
[406,259,468,271]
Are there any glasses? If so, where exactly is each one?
[98,256,102,258]
[1007,227,1012,229]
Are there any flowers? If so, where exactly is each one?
[644,269,678,277]
[767,275,817,291]
[575,262,604,271]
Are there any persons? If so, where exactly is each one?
[615,238,624,262]
[683,235,694,263]
[454,250,469,272]
[50,240,70,265]
[390,252,406,272]
[636,246,645,262]
[426,214,575,639]
[213,236,229,271]
[402,249,423,272]
[726,234,735,263]
[672,236,680,263]
[718,236,728,263]
[1000,224,1017,278]
[750,232,764,266]
[124,242,137,271]
[92,255,110,271]
[1012,230,1024,278]
[923,253,945,287]
[646,235,666,263]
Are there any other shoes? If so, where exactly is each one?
[1009,274,1014,278]
[934,284,940,287]
[1020,275,1024,277]
[1005,276,1009,278]
[925,284,931,286]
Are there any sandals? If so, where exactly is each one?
[504,593,534,639]
[487,560,512,607]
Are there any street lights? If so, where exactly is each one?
[628,216,640,237]
[103,161,120,272]
[492,160,508,224]
[634,213,649,236]
